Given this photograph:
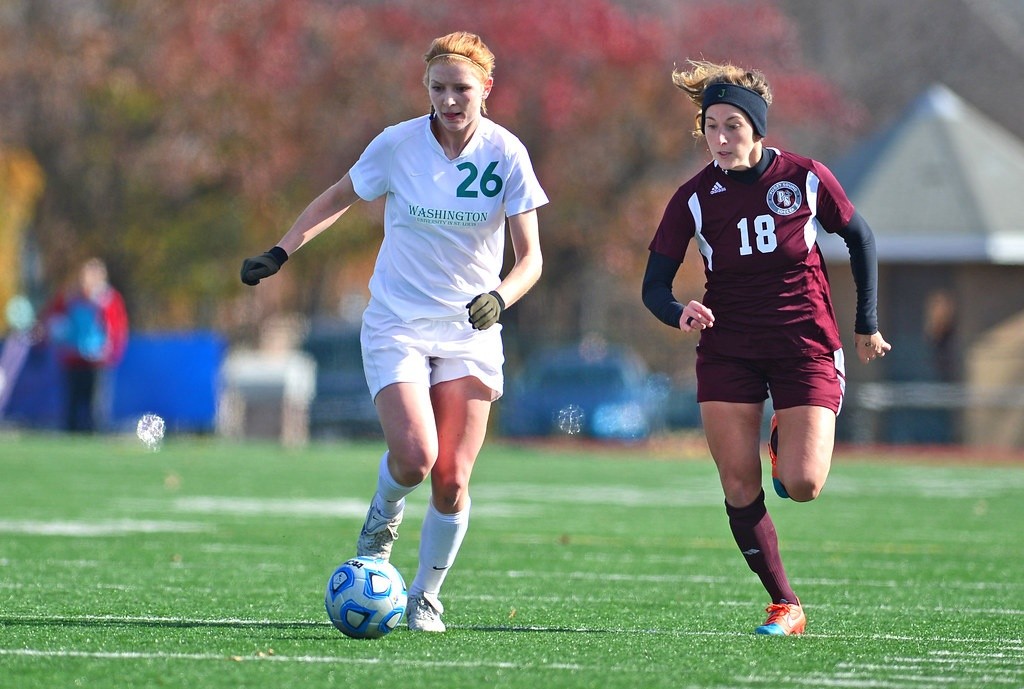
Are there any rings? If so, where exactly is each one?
[687,317,693,325]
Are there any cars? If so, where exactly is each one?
[499,347,670,440]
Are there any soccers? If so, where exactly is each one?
[323,555,409,641]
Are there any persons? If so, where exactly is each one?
[42,257,129,433]
[641,63,891,642]
[241,32,550,632]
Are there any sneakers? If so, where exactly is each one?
[407,591,446,633]
[356,493,404,563]
[768,412,792,498]
[754,591,806,634]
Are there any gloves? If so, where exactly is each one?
[240,246,287,286]
[466,291,505,331]
